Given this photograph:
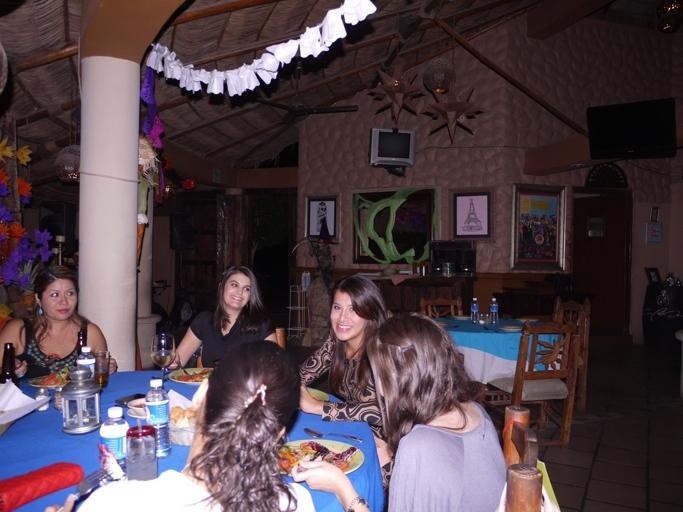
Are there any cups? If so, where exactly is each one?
[443,263,455,278]
[93,351,111,387]
[150,333,176,391]
[125,425,159,482]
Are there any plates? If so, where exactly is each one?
[126,409,148,419]
[307,388,330,401]
[167,367,214,386]
[453,316,472,321]
[28,383,68,388]
[518,319,538,322]
[498,327,523,332]
[281,439,365,477]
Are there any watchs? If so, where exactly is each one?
[342,495,368,511]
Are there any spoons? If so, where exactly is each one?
[303,427,364,444]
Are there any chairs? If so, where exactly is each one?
[504,404,543,512]
[487,318,582,453]
[568,308,592,424]
[420,297,463,317]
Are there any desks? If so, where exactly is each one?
[372,273,466,312]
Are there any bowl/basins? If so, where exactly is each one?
[125,397,148,416]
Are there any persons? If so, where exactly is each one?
[167,267,278,369]
[45,339,314,512]
[0,265,118,379]
[292,313,506,511]
[297,275,394,488]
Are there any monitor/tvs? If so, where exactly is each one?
[371,128,415,166]
[585,98,676,161]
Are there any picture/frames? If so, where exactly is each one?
[646,266,661,285]
[453,190,491,240]
[304,194,342,244]
[511,183,568,275]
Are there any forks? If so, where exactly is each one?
[310,447,330,462]
[55,466,114,512]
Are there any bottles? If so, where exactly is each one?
[100,406,129,471]
[126,426,156,480]
[471,298,479,324]
[146,379,170,457]
[489,298,498,326]
[77,347,96,381]
[0,343,19,388]
